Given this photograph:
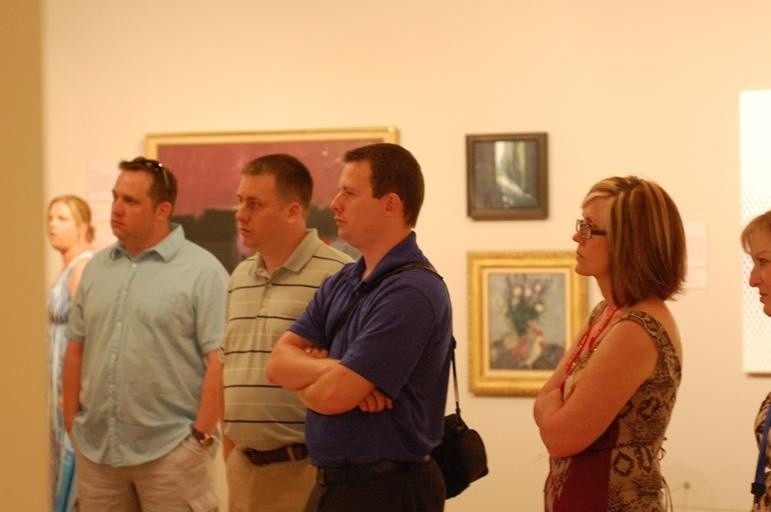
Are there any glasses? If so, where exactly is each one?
[131,156,170,192]
[574,217,607,240]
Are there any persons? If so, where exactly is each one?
[61,156,230,512]
[739,210,771,512]
[221,152,357,511]
[532,175,687,511]
[265,142,453,512]
[45,196,95,511]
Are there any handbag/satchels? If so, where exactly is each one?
[431,414,488,499]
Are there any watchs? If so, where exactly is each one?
[188,423,216,448]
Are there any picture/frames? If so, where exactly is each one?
[140,126,400,278]
[464,248,591,399]
[465,131,550,222]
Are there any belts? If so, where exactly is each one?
[242,442,307,467]
[315,454,430,486]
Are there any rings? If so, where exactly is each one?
[305,349,312,352]
